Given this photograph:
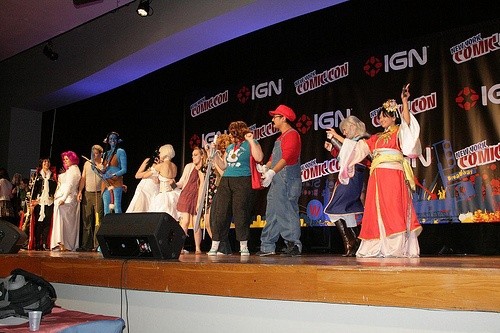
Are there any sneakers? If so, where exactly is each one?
[281,245,300,256]
[255,249,276,256]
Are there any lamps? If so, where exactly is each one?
[43,40,59,61]
[135,0,153,17]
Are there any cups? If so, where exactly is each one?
[28,311,42,332]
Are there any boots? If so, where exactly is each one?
[335,219,356,257]
[351,224,362,255]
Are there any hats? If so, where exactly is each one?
[268,104,296,121]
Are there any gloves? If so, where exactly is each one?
[261,169,275,187]
[261,165,269,175]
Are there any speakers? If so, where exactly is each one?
[96,211,187,260]
[0,220,28,255]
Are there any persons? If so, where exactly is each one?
[49,151,81,252]
[75,144,103,252]
[96,132,127,252]
[257,104,303,257]
[326,83,422,257]
[126,121,263,256]
[0,158,57,251]
[324,115,372,256]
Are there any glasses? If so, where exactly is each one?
[273,115,284,119]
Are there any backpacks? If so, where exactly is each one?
[0,268,57,325]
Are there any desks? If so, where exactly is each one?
[0,306,122,333]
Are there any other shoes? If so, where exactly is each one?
[208,250,216,255]
[195,250,201,254]
[240,247,250,256]
[206,250,233,256]
[38,244,45,251]
[76,248,92,252]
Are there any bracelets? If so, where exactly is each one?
[112,173,116,176]
